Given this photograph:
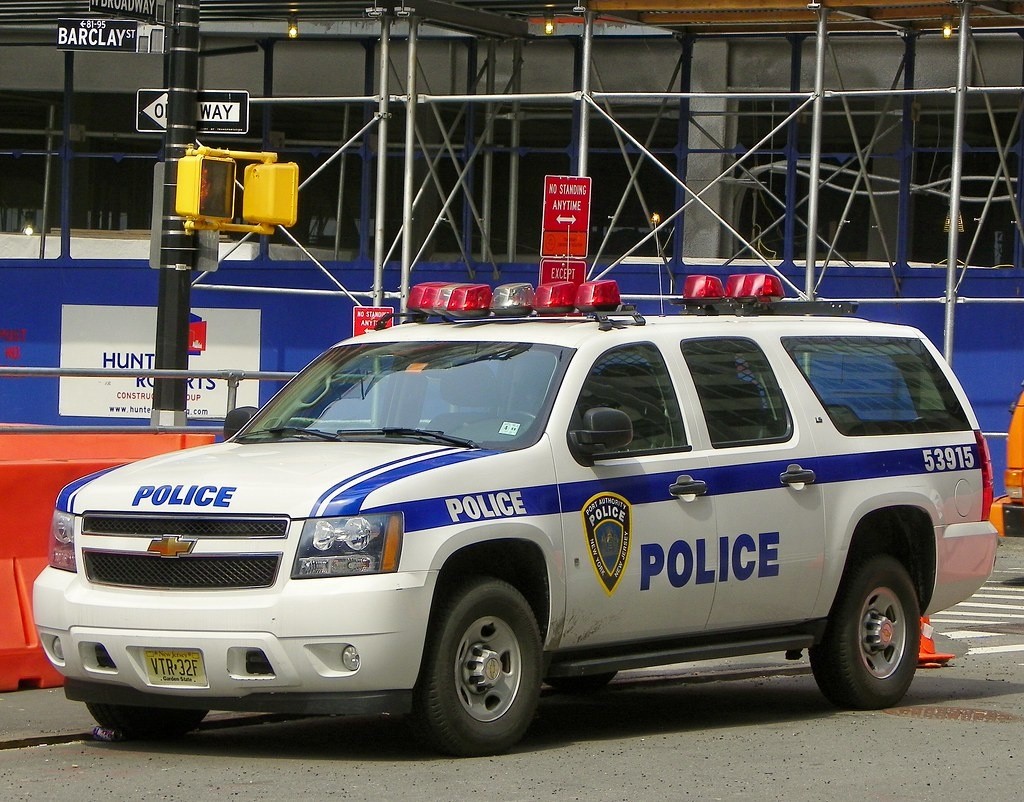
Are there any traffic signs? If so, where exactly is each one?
[135,84,250,142]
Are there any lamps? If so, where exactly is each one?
[942,16,953,39]
[288,14,299,39]
[543,9,555,36]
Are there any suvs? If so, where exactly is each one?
[30,270,1004,759]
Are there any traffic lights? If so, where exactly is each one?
[177,156,240,225]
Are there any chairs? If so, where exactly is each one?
[427,363,504,428]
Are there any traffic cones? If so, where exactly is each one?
[920,616,957,668]
